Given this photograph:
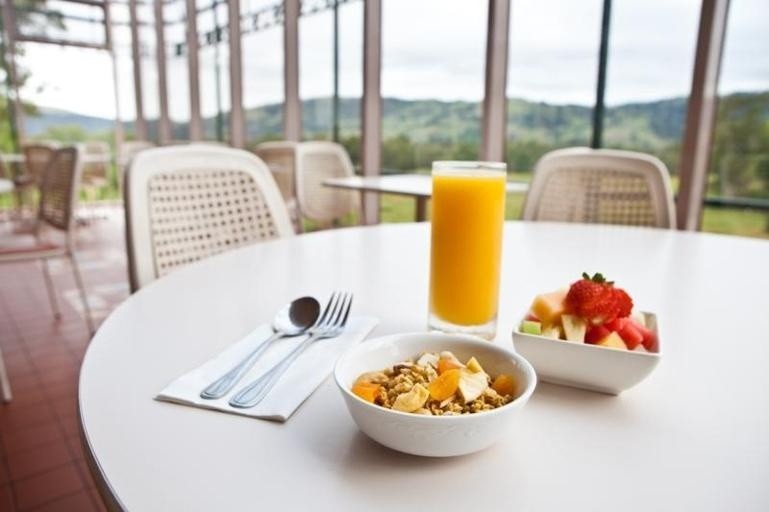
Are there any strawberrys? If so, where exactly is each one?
[567,272,634,326]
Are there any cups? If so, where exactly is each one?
[426,158,509,343]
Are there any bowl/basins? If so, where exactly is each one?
[330,330,540,460]
[511,291,663,397]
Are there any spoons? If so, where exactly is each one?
[198,296,322,401]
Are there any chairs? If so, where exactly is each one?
[294,141,364,229]
[2,143,99,341]
[112,140,149,190]
[123,144,294,300]
[3,143,88,233]
[521,150,678,232]
[531,144,594,226]
[251,141,307,233]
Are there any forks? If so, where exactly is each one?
[227,290,355,411]
